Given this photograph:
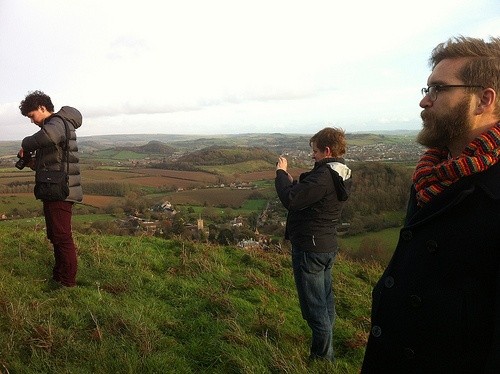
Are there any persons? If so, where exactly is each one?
[274,125,356,363]
[364,35,499,374]
[13,91,86,289]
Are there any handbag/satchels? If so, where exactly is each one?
[33,170,70,202]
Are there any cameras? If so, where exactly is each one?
[15,152,32,169]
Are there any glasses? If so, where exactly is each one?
[421,84,488,103]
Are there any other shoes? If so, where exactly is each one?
[41,280,65,293]
